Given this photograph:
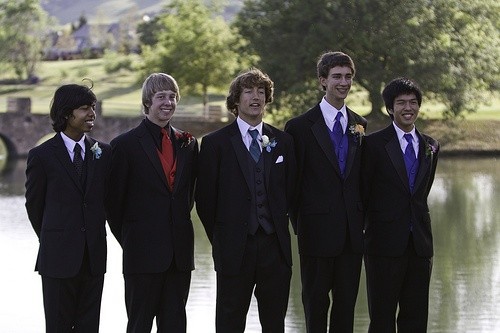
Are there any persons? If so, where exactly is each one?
[108,74,197,333]
[284,52,367,333]
[194,71,292,333]
[25,84,112,333]
[361,78,440,333]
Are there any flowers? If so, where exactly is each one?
[90,141,102,161]
[348,121,367,146]
[261,135,277,153]
[176,127,194,149]
[425,140,437,161]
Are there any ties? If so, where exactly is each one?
[247,128,261,162]
[404,132,417,166]
[161,127,174,184]
[334,111,344,141]
[73,142,83,183]
